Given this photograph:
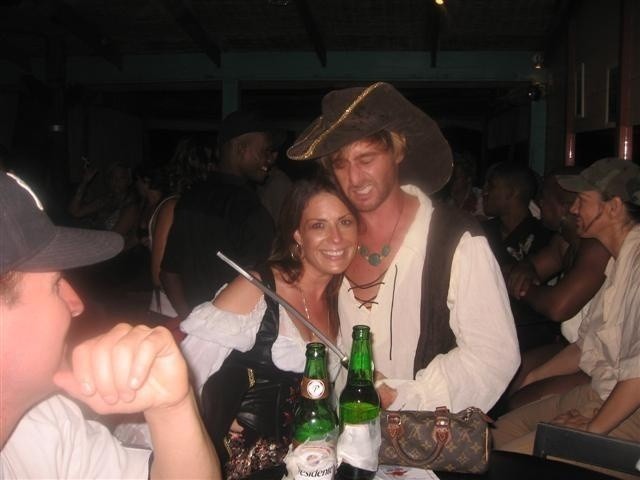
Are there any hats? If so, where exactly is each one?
[286,80,455,196]
[0,165,125,278]
[554,156,640,205]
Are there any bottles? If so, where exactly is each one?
[337,326,381,479]
[292,342,337,480]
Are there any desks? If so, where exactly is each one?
[235,450,620,478]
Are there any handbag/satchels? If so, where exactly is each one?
[379,406,496,475]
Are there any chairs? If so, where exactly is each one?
[534,419,640,479]
